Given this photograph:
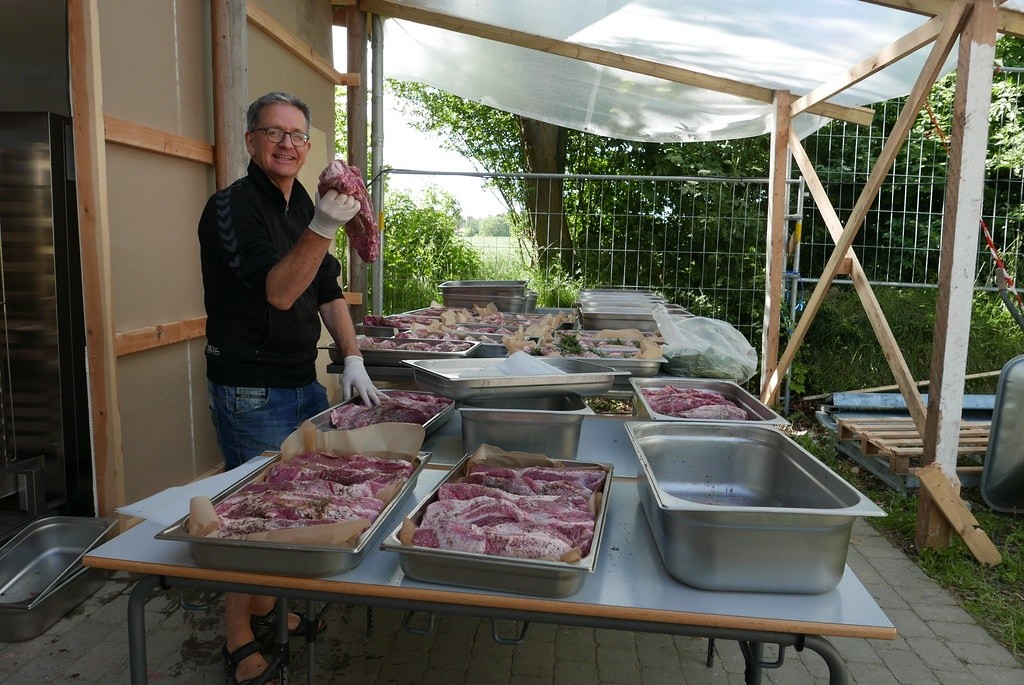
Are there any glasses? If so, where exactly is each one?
[249,127,310,148]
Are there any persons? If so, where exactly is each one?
[198,90,389,685]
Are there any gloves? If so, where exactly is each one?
[308,190,360,240]
[342,356,389,407]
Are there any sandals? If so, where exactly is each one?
[222,600,327,685]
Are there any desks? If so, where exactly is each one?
[79,404,901,685]
